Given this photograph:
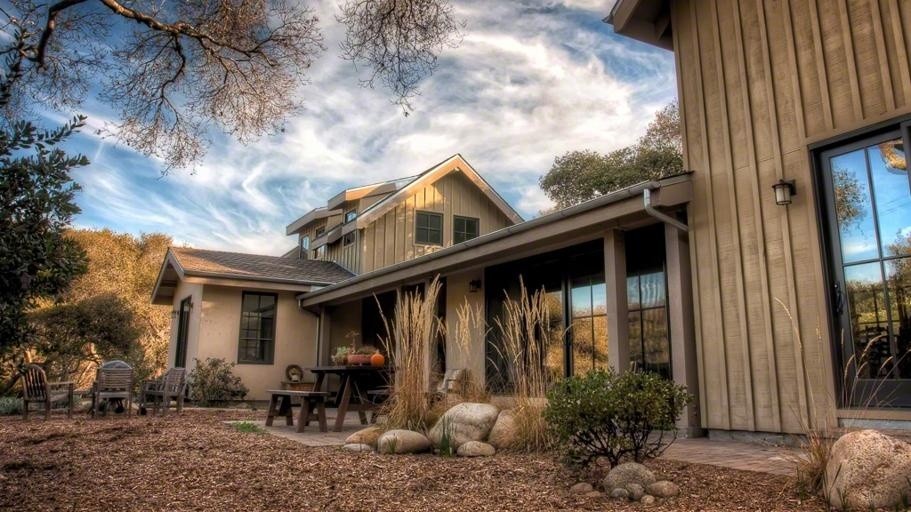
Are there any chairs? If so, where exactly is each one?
[91,360,187,420]
[20,364,74,423]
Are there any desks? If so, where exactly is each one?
[304,364,389,432]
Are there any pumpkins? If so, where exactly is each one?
[369,348,385,366]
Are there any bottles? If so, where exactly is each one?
[370,349,384,368]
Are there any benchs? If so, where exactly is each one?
[265,388,432,433]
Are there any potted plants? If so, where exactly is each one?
[334,327,376,365]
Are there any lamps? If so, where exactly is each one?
[770,177,799,206]
